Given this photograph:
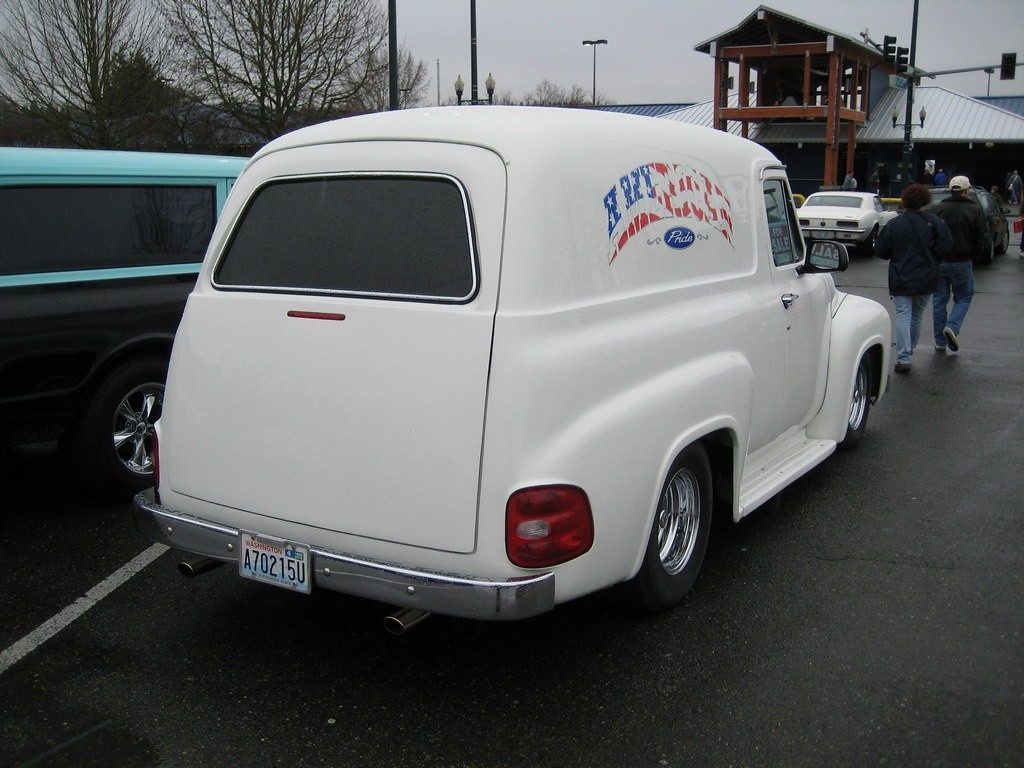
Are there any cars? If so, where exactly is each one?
[136,105,894,635]
[796,191,899,259]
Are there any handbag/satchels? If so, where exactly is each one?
[1008,184,1013,191]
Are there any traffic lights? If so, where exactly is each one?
[896,47,910,74]
[883,35,901,67]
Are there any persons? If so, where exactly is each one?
[931,176,987,351]
[1019,200,1024,253]
[991,186,1003,206]
[877,184,952,371]
[1005,171,1022,204]
[871,170,879,191]
[844,170,854,191]
[934,169,947,185]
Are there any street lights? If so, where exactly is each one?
[889,105,930,195]
[581,39,611,106]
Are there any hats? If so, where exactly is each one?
[949,176,973,192]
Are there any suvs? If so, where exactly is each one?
[921,185,1012,264]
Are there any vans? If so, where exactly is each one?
[0,148,270,512]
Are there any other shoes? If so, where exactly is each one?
[942,327,959,351]
[935,344,946,351]
[1013,202,1018,204]
[894,364,911,373]
[1008,199,1012,203]
